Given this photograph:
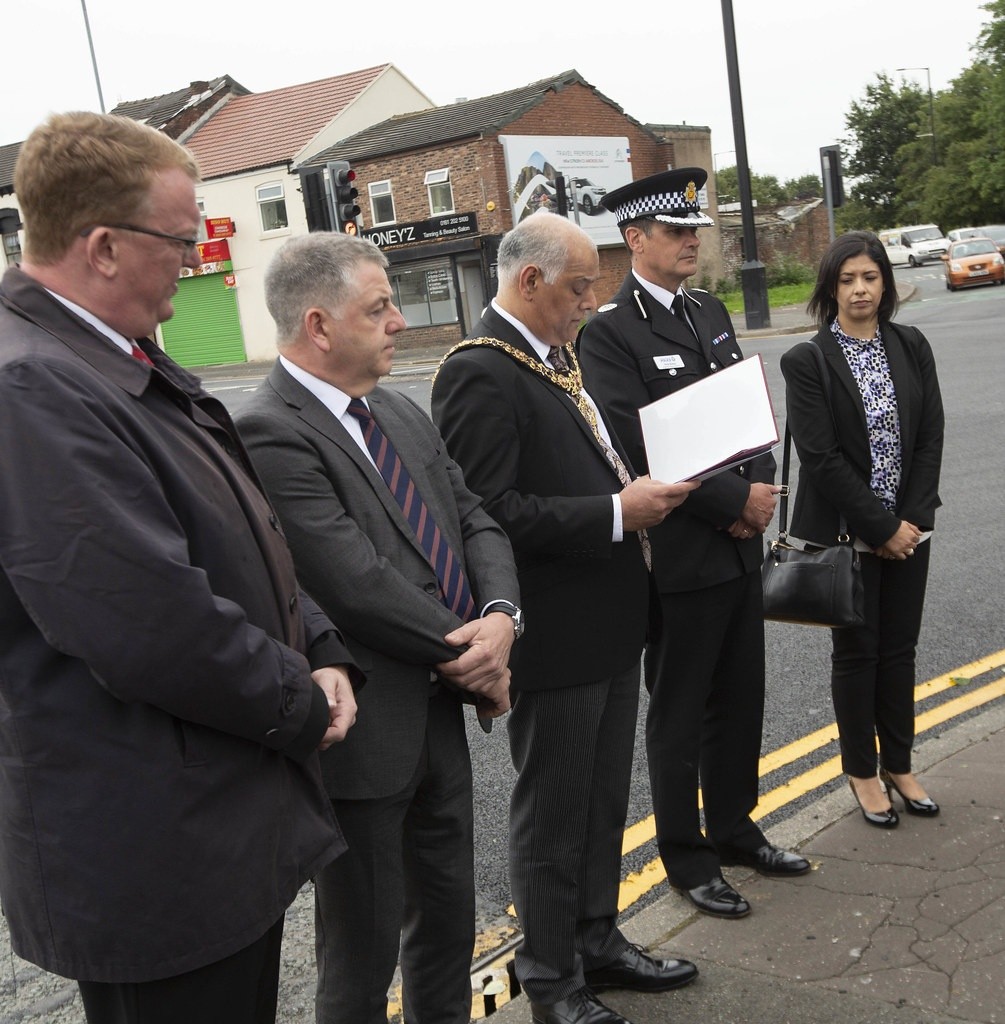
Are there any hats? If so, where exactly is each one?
[600,166,716,228]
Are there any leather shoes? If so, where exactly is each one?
[587,942,699,991]
[670,875,752,919]
[529,992,630,1024]
[717,840,812,879]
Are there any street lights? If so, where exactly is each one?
[896,68,937,167]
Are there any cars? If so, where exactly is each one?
[941,238,1004,292]
[948,225,1005,257]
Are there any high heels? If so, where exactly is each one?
[847,768,942,827]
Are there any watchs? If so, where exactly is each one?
[484,600,525,640]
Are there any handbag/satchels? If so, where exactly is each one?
[761,542,865,627]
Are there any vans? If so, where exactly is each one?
[879,224,952,268]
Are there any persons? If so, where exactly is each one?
[778,226,947,830]
[229,230,527,1024]
[599,166,813,923]
[0,108,368,1024]
[426,206,703,1024]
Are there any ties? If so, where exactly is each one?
[671,296,695,339]
[343,397,477,626]
[548,347,652,573]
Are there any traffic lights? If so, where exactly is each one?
[327,160,362,239]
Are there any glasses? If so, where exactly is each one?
[79,225,200,258]
